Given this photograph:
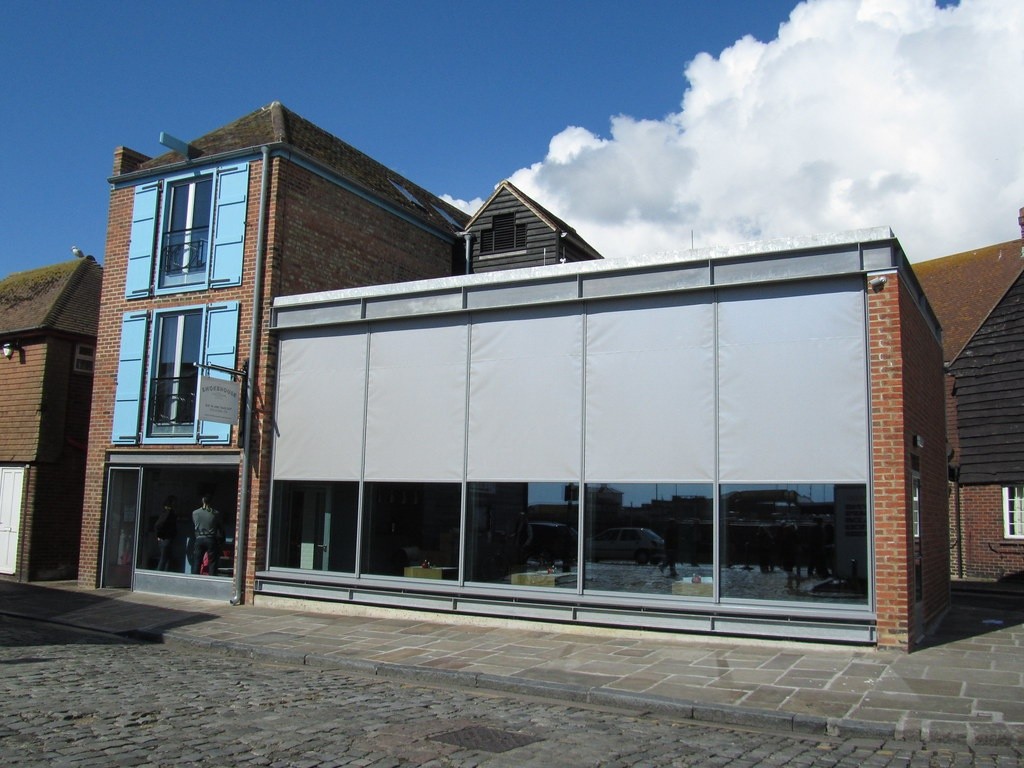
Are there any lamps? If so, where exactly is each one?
[2,338,24,360]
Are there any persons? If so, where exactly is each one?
[508,512,533,565]
[658,513,685,576]
[684,520,703,567]
[758,516,834,597]
[191,494,223,576]
[154,495,178,571]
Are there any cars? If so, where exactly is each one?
[584,527,665,564]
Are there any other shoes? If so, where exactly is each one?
[658,565,665,574]
[669,572,677,577]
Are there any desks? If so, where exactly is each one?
[511,570,577,589]
[404,566,458,581]
[672,577,713,597]
